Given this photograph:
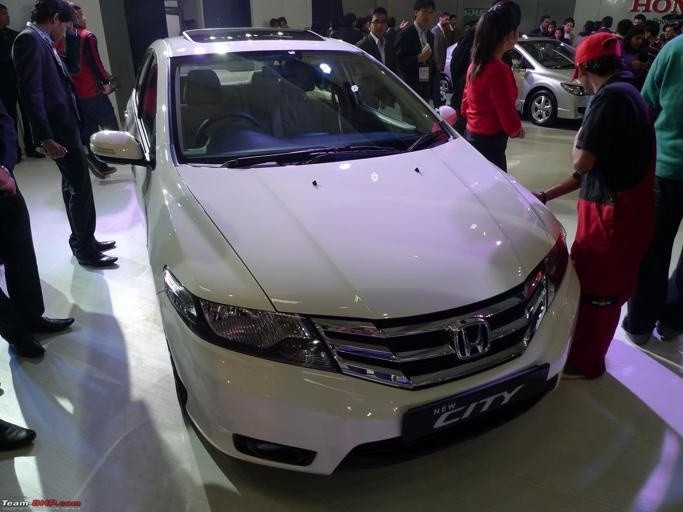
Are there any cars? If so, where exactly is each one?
[438,36,593,126]
[88,26,580,478]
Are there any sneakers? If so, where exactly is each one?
[103,163,117,174]
[85,154,105,179]
[561,361,585,381]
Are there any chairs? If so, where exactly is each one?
[179,65,360,151]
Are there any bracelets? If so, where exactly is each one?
[539,189,547,206]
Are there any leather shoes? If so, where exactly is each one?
[78,253,118,267]
[98,241,116,250]
[0,419,37,452]
[10,336,46,358]
[26,151,45,158]
[15,153,22,163]
[37,316,74,334]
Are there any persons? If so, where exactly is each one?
[447,14,459,45]
[622,28,683,346]
[270,17,303,66]
[430,12,454,108]
[616,13,680,92]
[393,3,434,125]
[572,16,615,48]
[0,3,120,179]
[460,2,525,174]
[528,15,575,64]
[0,383,36,457]
[450,23,478,135]
[10,1,121,268]
[324,7,409,109]
[0,102,75,359]
[532,33,657,379]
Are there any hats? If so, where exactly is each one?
[570,32,621,82]
